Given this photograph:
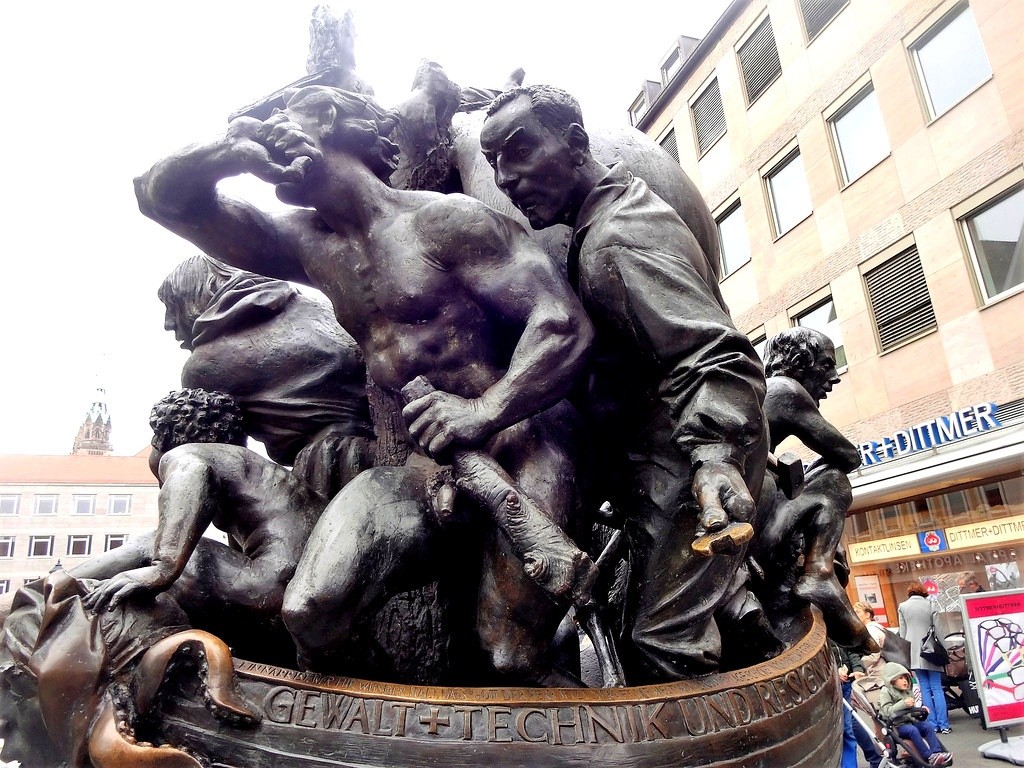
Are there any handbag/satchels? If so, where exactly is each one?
[920,612,949,667]
[873,624,910,670]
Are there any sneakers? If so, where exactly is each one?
[934,729,937,733]
[930,752,952,765]
[940,728,952,734]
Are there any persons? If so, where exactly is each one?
[826,635,866,768]
[958,569,990,730]
[897,584,953,734]
[878,661,952,766]
[52,57,881,687]
[850,601,901,746]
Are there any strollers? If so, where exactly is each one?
[839,667,953,768]
